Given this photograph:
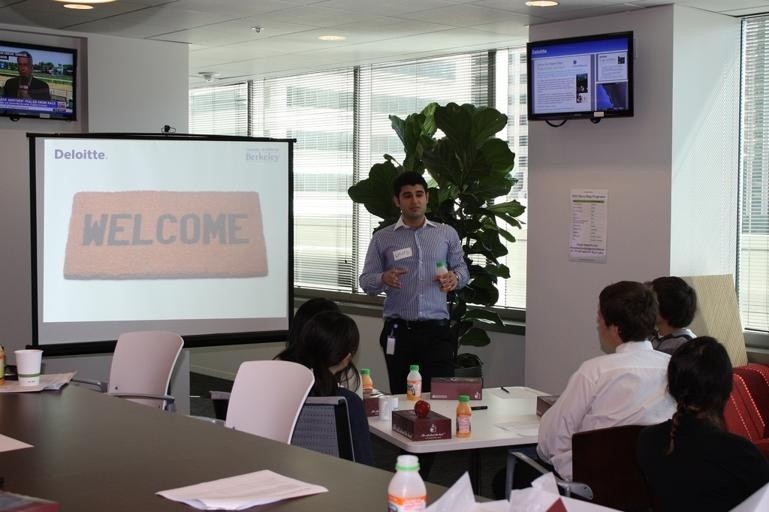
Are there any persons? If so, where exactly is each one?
[273,312,360,397]
[595,336,769,512]
[0,51,50,118]
[358,171,469,394]
[287,298,360,392]
[492,281,678,500]
[650,276,696,356]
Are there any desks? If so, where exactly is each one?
[368,386,554,500]
[0,383,498,512]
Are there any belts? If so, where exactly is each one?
[404,319,448,330]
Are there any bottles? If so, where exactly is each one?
[455,394,473,439]
[406,364,422,400]
[359,368,373,397]
[436,261,451,292]
[387,454,427,512]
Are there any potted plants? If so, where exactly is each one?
[348,101,525,388]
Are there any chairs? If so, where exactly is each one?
[105,331,185,410]
[502,425,659,512]
[208,389,357,463]
[224,360,315,445]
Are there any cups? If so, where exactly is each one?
[378,396,399,420]
[14,349,43,387]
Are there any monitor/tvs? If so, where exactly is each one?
[0,38,78,125]
[526,30,635,122]
